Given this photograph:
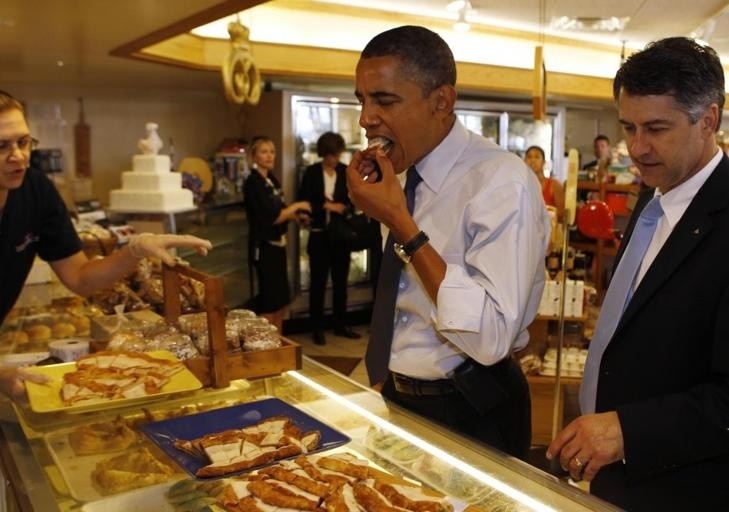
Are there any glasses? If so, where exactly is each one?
[0,136,39,155]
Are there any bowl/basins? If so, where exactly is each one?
[31,149,62,173]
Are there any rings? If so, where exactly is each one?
[575,455,585,468]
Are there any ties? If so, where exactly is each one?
[366,165,426,387]
[578,193,664,413]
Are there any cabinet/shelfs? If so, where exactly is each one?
[101,192,262,316]
[524,303,587,448]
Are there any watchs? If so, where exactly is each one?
[394,230,431,264]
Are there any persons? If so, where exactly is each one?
[547,35,727,511]
[299,131,363,343]
[343,27,549,456]
[523,143,568,219]
[243,134,313,338]
[580,136,614,168]
[0,89,212,325]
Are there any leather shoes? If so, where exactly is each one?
[308,324,361,345]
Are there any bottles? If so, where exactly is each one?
[213,137,247,202]
[72,96,91,179]
[548,248,587,280]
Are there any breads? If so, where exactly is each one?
[362,137,391,160]
[0,226,455,512]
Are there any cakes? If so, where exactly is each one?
[109,122,194,214]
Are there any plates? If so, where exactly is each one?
[138,397,352,480]
[22,351,203,415]
